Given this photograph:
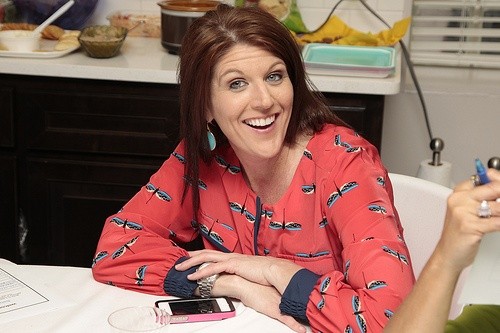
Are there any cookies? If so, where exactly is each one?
[0,23,65,39]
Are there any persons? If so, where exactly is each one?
[92,4,416,333]
[382,168,500,333]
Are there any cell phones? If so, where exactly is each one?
[155,296,236,324]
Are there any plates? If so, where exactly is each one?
[0,23,82,59]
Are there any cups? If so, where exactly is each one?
[107,305,171,332]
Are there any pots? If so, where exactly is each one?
[157,1,223,54]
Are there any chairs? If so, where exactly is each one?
[387,174,500,319]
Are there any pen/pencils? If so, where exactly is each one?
[475,158,489,185]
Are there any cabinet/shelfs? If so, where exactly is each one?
[0,72,386,266]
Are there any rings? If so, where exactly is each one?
[478,200,492,218]
[471,174,481,185]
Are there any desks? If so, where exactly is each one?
[1,264,296,333]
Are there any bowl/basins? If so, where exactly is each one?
[0,30,42,52]
[78,25,128,58]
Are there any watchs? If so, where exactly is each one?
[196,262,220,298]
